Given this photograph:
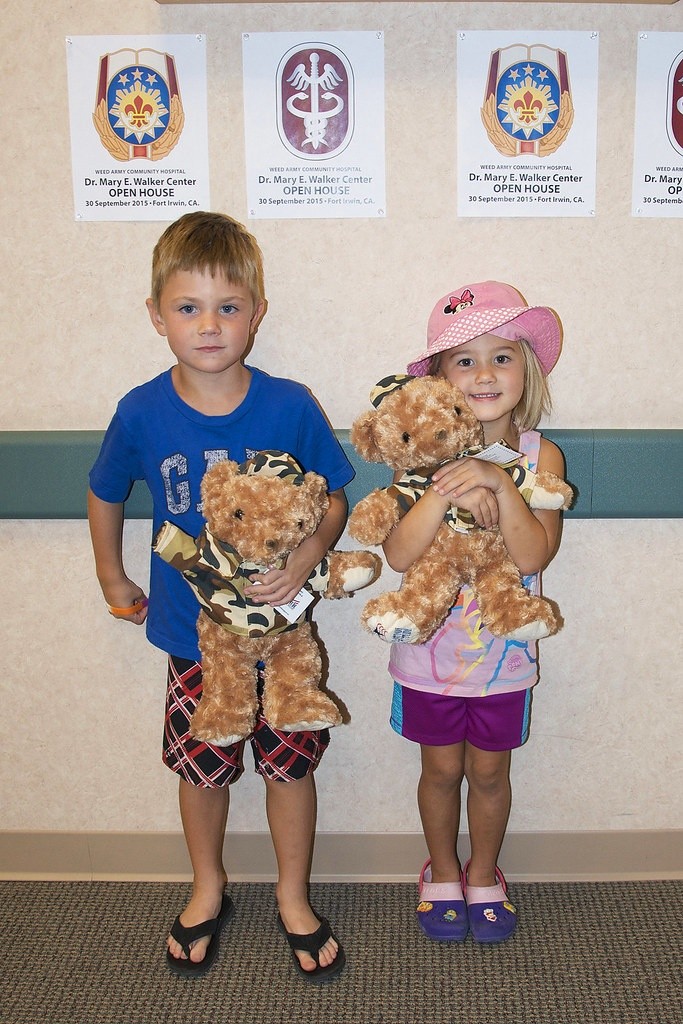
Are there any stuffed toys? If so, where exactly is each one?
[155,444,386,747]
[347,375,560,648]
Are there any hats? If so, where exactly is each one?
[406,279,563,380]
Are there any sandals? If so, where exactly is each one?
[417,856,469,941]
[462,857,517,944]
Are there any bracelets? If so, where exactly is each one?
[106,597,150,616]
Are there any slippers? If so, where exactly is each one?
[167,893,235,976]
[275,907,347,982]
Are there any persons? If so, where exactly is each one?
[87,213,358,982]
[380,280,566,953]
